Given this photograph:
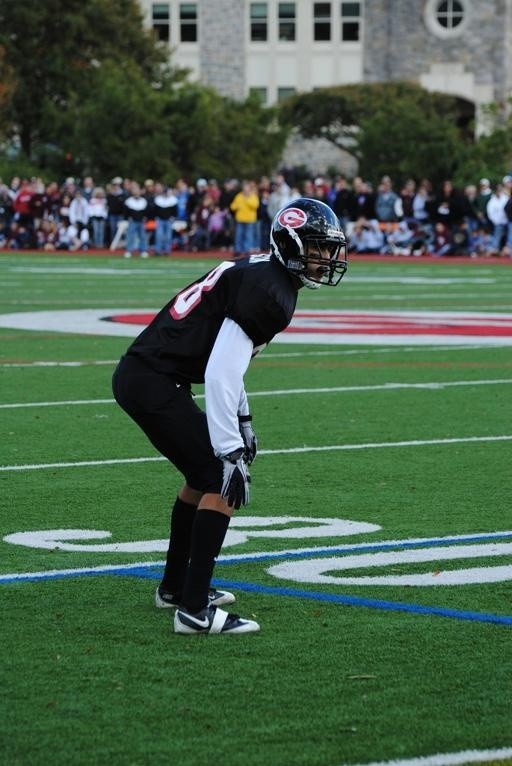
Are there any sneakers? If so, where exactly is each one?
[154,583,236,609]
[173,605,261,635]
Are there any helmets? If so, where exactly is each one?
[268,196,348,278]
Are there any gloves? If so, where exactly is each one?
[238,415,259,466]
[218,447,253,511]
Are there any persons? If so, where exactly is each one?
[1,171,511,258]
[108,193,353,639]
